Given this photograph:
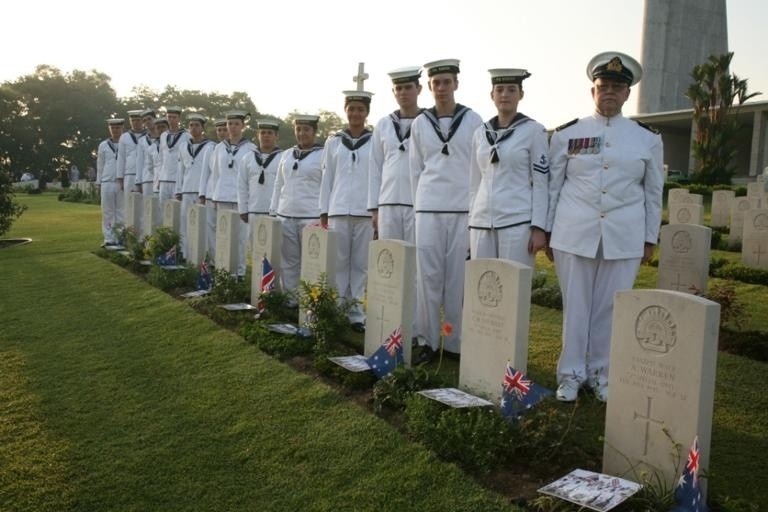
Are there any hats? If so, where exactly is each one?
[257,118,280,131]
[489,68,531,85]
[107,107,206,127]
[293,113,320,129]
[386,58,423,84]
[214,109,249,127]
[586,51,643,86]
[343,91,374,104]
[424,59,460,76]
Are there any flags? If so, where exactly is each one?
[674,433,703,512]
[500,363,553,424]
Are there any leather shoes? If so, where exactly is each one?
[351,323,365,333]
[413,344,438,364]
[557,382,578,401]
[593,384,607,401]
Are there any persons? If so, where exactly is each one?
[39,170,47,191]
[70,165,80,182]
[21,167,34,182]
[60,165,71,193]
[85,165,96,181]
